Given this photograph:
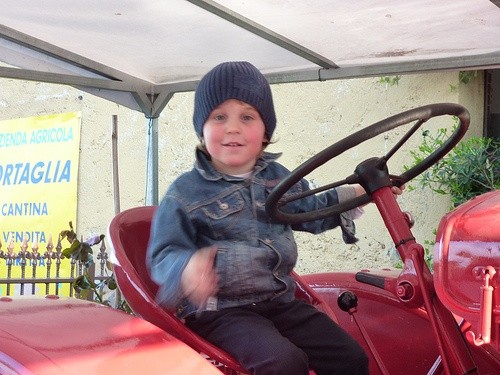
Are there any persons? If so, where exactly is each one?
[146,62,405,375]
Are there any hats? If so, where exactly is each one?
[193,60,276,148]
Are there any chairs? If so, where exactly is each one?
[106,206,338,375]
